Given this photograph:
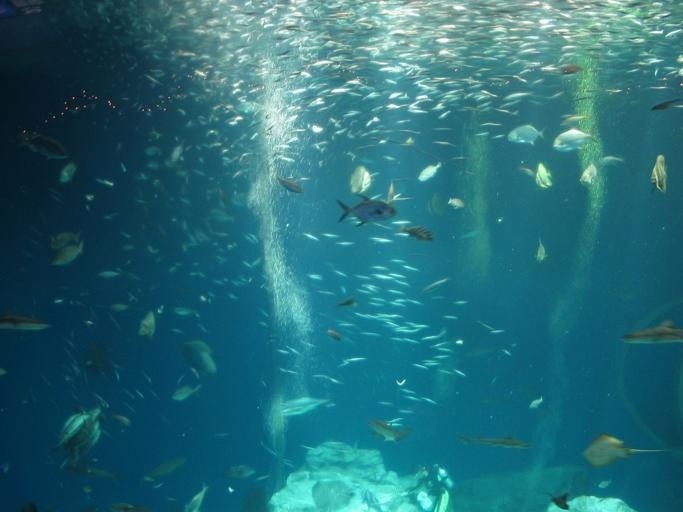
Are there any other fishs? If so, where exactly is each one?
[0,1,682,511]
